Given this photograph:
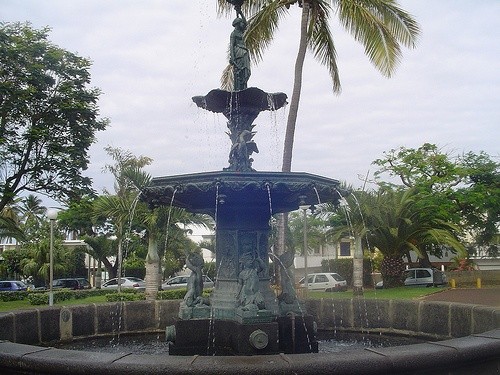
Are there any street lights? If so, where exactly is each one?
[47,206,58,307]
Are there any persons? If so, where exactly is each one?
[235,252,265,305]
[280,253,296,295]
[185,245,204,297]
[230,6,251,88]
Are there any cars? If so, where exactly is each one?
[101,277,146,289]
[30,279,91,291]
[0,280,27,291]
[162,275,216,290]
[298,273,347,293]
[376,267,446,287]
